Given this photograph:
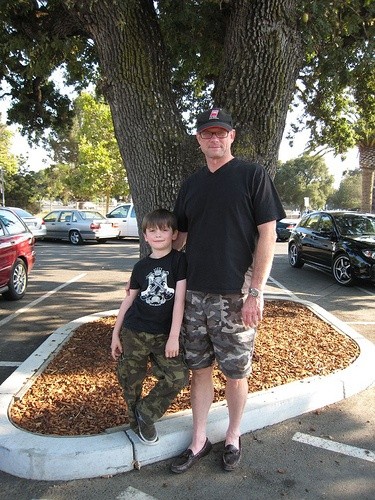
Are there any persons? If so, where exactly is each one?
[110,208,187,446]
[171,108,287,473]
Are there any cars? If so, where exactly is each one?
[275,219,297,242]
[41,209,122,246]
[4,206,48,242]
[287,211,375,287]
[105,202,140,240]
[0,206,36,301]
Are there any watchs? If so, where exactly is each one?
[248,288,263,297]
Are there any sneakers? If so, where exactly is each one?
[130,408,160,446]
[222,436,243,471]
[170,437,212,474]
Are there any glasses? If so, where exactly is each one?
[200,129,229,139]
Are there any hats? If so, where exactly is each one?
[196,107,233,133]
